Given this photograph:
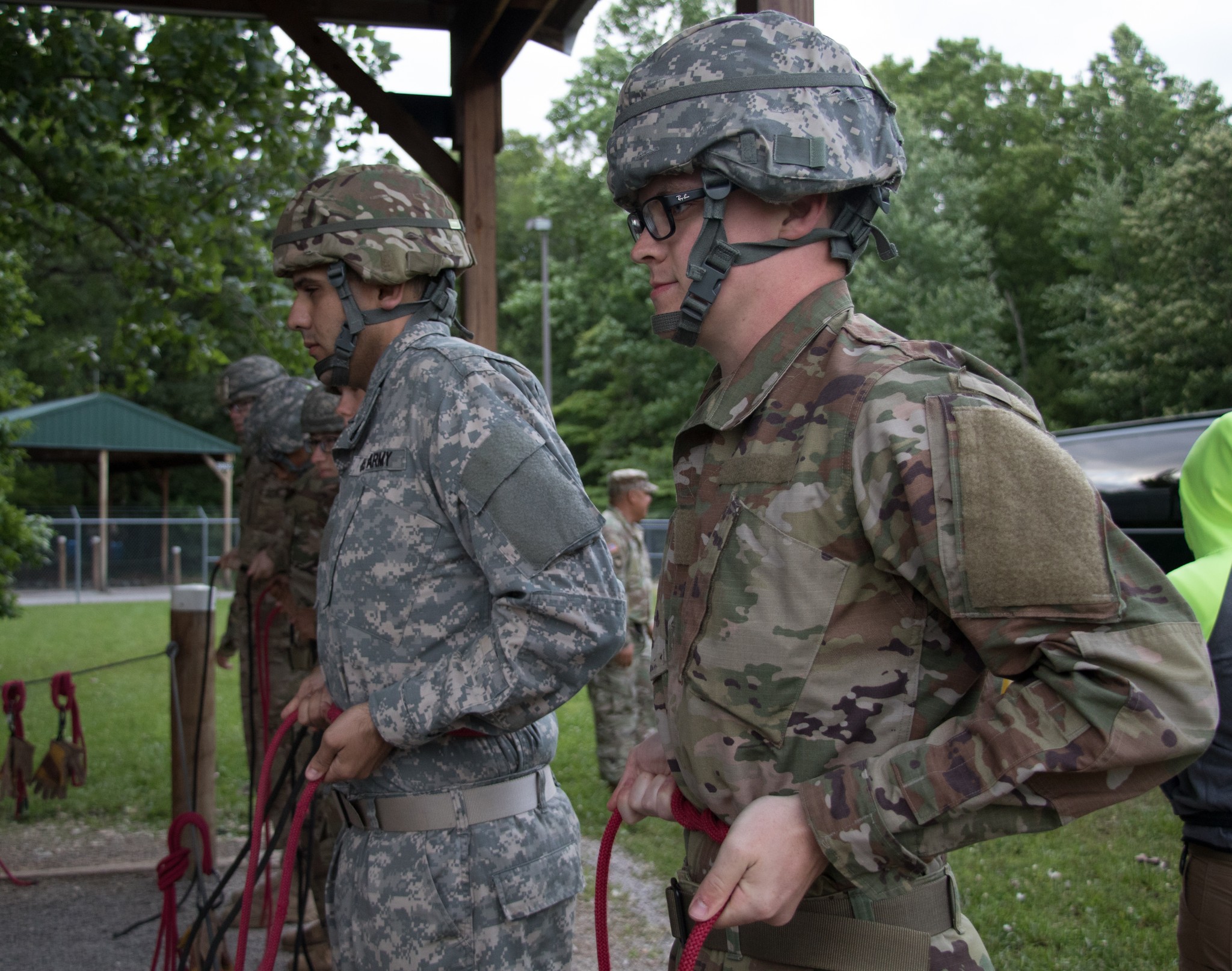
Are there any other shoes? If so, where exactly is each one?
[208,867,329,928]
[281,912,335,970]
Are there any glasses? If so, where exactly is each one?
[626,188,707,242]
[302,436,338,453]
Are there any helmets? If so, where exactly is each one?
[243,378,322,461]
[216,355,291,405]
[271,164,477,284]
[298,383,346,433]
[606,9,906,209]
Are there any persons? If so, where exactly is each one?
[586,468,657,792]
[216,348,365,971]
[1160,411,1232,971]
[605,8,1222,971]
[279,164,626,971]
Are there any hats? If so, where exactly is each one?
[608,469,660,492]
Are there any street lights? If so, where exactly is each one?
[523,218,555,407]
